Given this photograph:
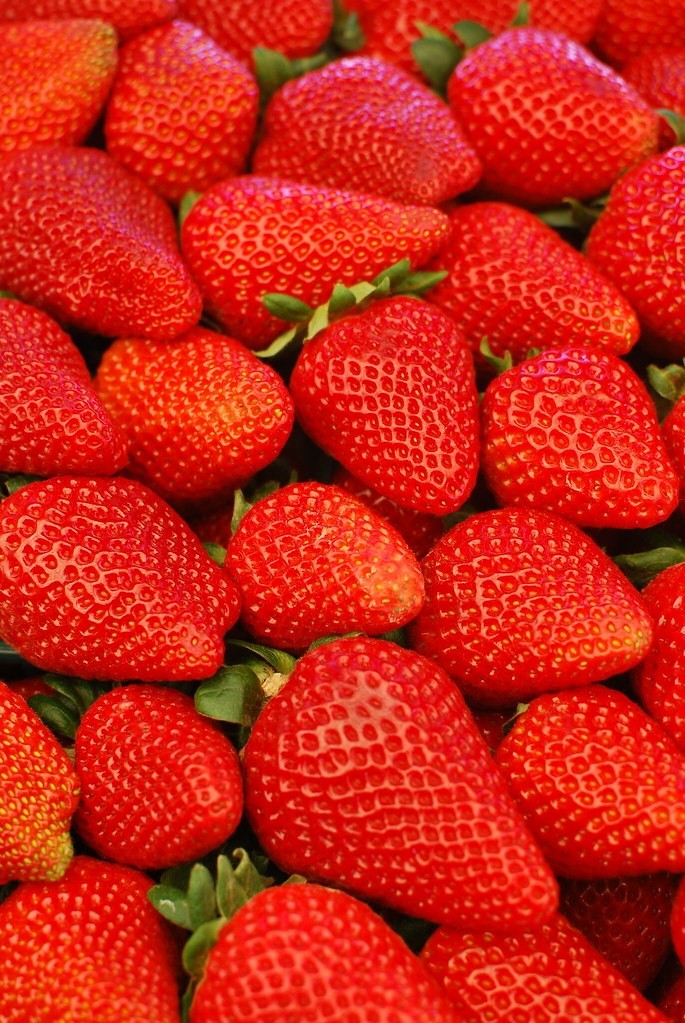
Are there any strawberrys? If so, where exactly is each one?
[0,0,685,1023]
